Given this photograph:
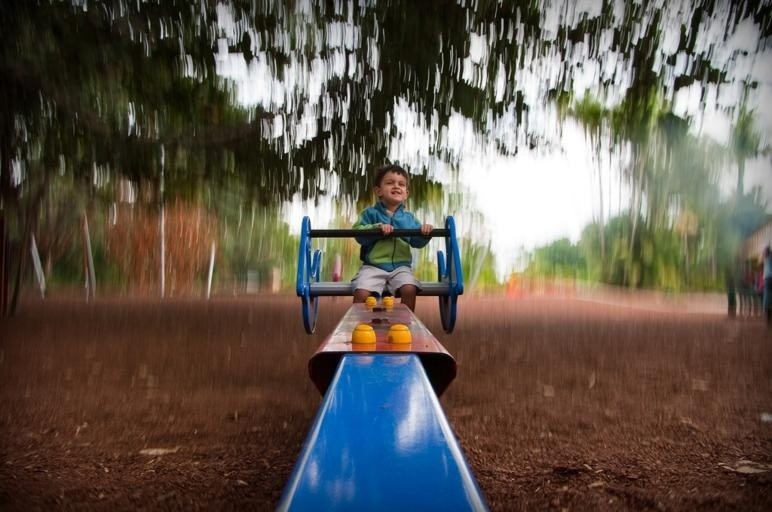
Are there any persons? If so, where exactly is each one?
[724,245,772,320]
[350,165,434,313]
